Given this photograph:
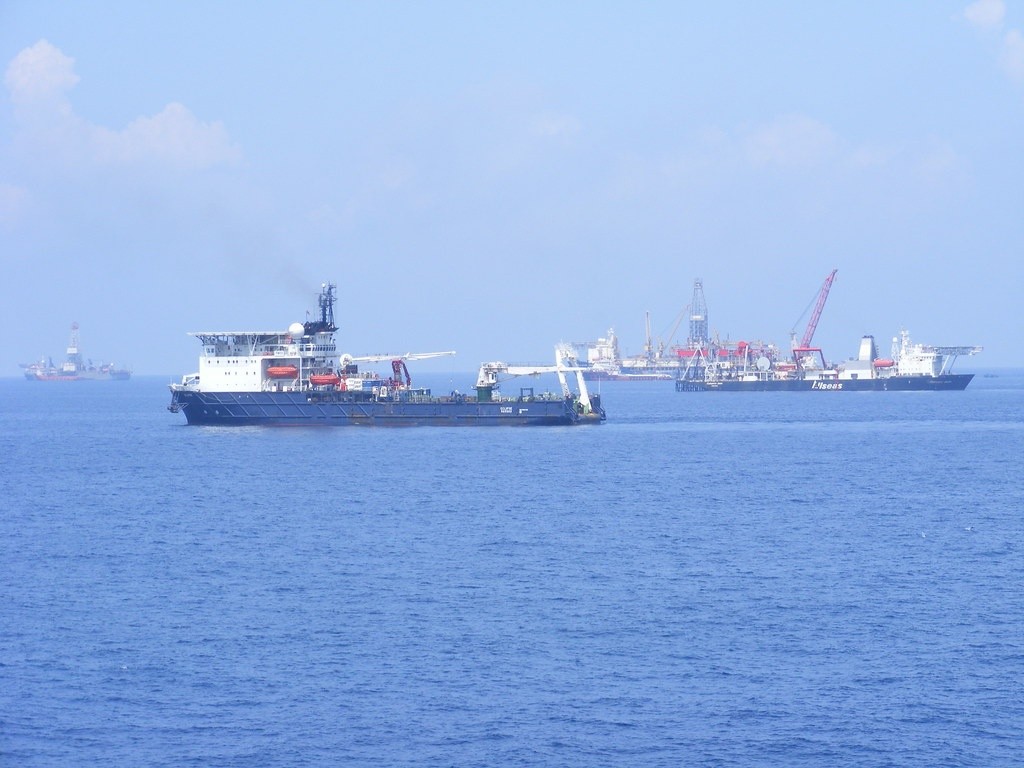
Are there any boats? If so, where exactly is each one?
[167,282,607,425]
[568,276,780,381]
[676,269,984,392]
[25,322,133,380]
